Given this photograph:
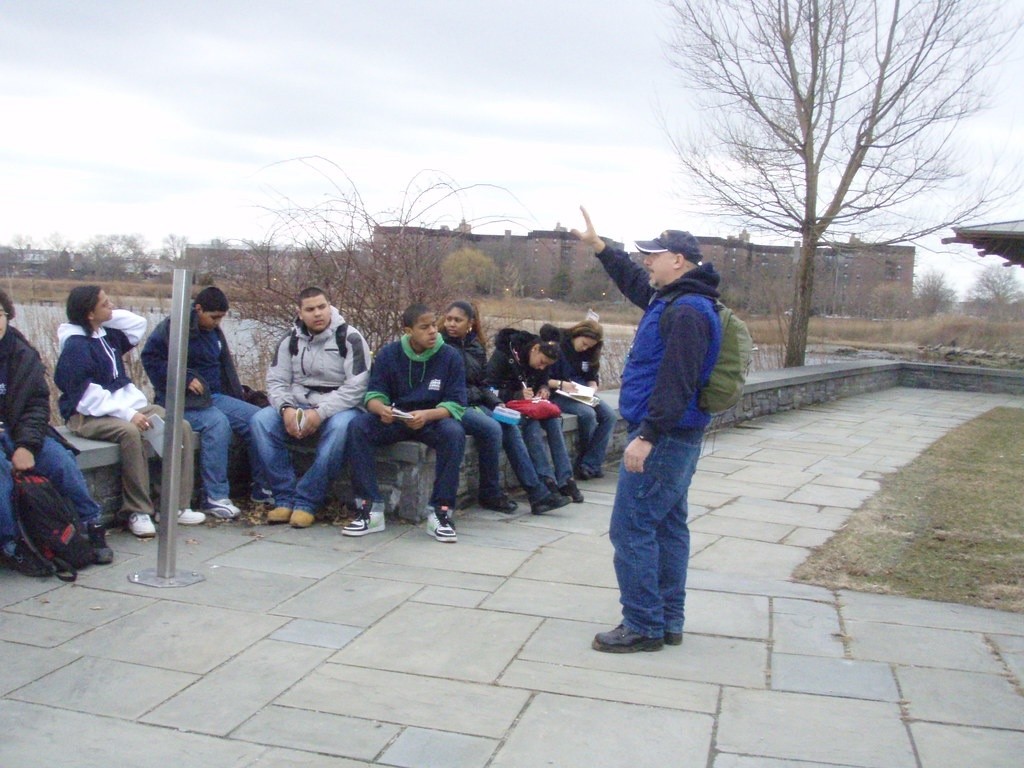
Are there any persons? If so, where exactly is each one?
[141,287,275,518]
[487,323,584,503]
[54,285,206,535]
[571,205,720,654]
[0,291,113,577]
[250,287,371,527]
[436,301,573,515]
[548,320,616,480]
[341,303,467,542]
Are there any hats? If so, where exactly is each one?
[634,230,703,265]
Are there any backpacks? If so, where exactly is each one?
[658,292,753,413]
[11,467,98,581]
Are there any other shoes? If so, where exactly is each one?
[530,493,573,515]
[290,508,314,527]
[4,542,55,577]
[558,483,583,502]
[267,506,294,524]
[88,522,113,564]
[546,480,561,494]
[480,493,518,514]
[574,464,605,480]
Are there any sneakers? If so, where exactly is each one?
[154,508,206,525]
[127,512,156,538]
[200,493,241,519]
[341,505,385,536]
[250,482,275,505]
[426,506,457,543]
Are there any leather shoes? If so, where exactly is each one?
[662,631,682,646]
[592,622,663,653]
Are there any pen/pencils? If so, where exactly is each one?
[391,403,395,410]
[145,421,149,427]
[569,377,572,383]
[521,380,533,400]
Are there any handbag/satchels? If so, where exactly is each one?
[505,399,561,419]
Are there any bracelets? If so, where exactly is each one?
[558,380,562,390]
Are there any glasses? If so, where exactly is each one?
[0,312,9,319]
